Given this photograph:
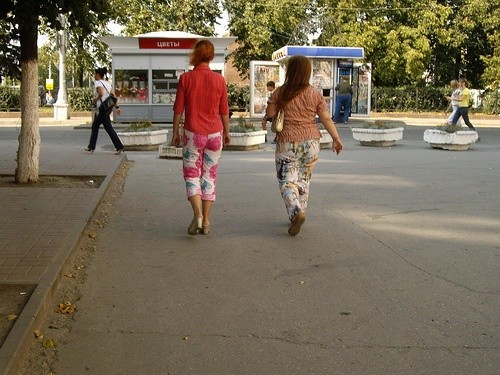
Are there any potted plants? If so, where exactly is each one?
[117,118,168,151]
[223,114,267,150]
[316,123,334,148]
[424,122,478,151]
[351,120,405,147]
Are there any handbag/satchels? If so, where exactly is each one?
[93,80,116,113]
[270,84,286,134]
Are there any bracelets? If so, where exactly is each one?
[115,106,119,108]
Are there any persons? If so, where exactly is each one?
[171,40,232,235]
[83,67,124,155]
[266,56,343,236]
[445,78,481,144]
[332,75,352,124]
[261,80,278,144]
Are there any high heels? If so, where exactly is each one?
[188,216,203,235]
[114,146,126,156]
[202,222,212,236]
[83,147,95,153]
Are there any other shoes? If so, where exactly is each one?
[271,136,277,144]
[287,210,306,236]
